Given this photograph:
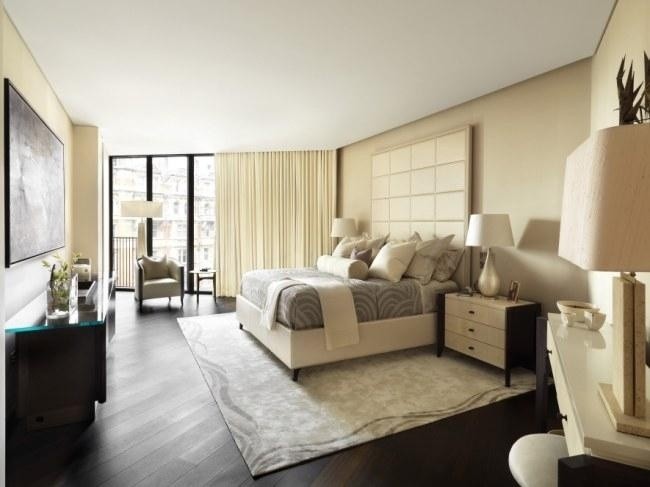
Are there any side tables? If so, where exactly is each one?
[189,270,217,304]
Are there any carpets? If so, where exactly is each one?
[176,312,554,479]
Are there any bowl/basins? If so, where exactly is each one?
[556,300,601,324]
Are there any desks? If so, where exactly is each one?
[5,280,108,437]
[529,311,650,487]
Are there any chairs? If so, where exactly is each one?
[136,256,184,314]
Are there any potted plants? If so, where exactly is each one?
[41,253,78,319]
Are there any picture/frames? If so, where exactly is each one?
[508,280,520,302]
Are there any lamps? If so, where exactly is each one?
[465,213,515,298]
[118,201,164,301]
[557,122,650,439]
[330,217,359,243]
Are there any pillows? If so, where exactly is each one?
[317,232,464,283]
[143,255,168,279]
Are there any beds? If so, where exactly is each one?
[236,243,472,382]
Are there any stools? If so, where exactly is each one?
[506,433,571,487]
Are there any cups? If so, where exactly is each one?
[583,310,607,331]
[560,311,576,328]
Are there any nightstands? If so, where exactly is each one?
[437,291,540,387]
